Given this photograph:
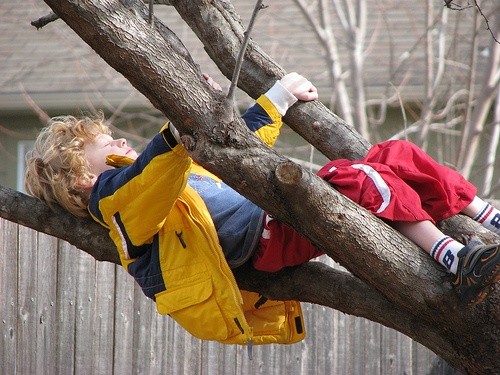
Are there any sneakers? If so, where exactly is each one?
[451,240,500,311]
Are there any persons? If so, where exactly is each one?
[26,70,500,346]
